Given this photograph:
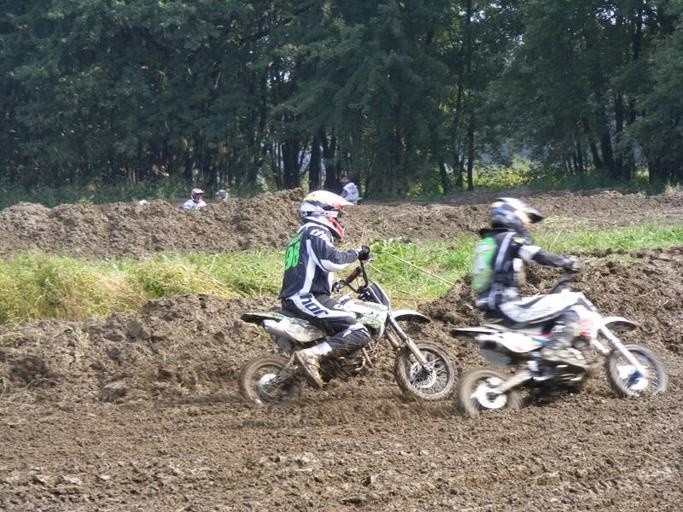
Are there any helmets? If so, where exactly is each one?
[338,175,349,187]
[300,189,354,238]
[214,190,229,202]
[488,197,544,231]
[191,188,204,203]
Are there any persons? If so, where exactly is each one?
[180,187,208,209]
[468,195,590,408]
[338,175,359,205]
[214,189,230,203]
[274,189,373,391]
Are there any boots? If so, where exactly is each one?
[542,332,588,369]
[295,341,335,390]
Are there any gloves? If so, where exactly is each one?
[563,254,580,273]
[357,245,370,260]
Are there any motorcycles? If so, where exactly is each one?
[450,255,670,414]
[238,244,458,404]
